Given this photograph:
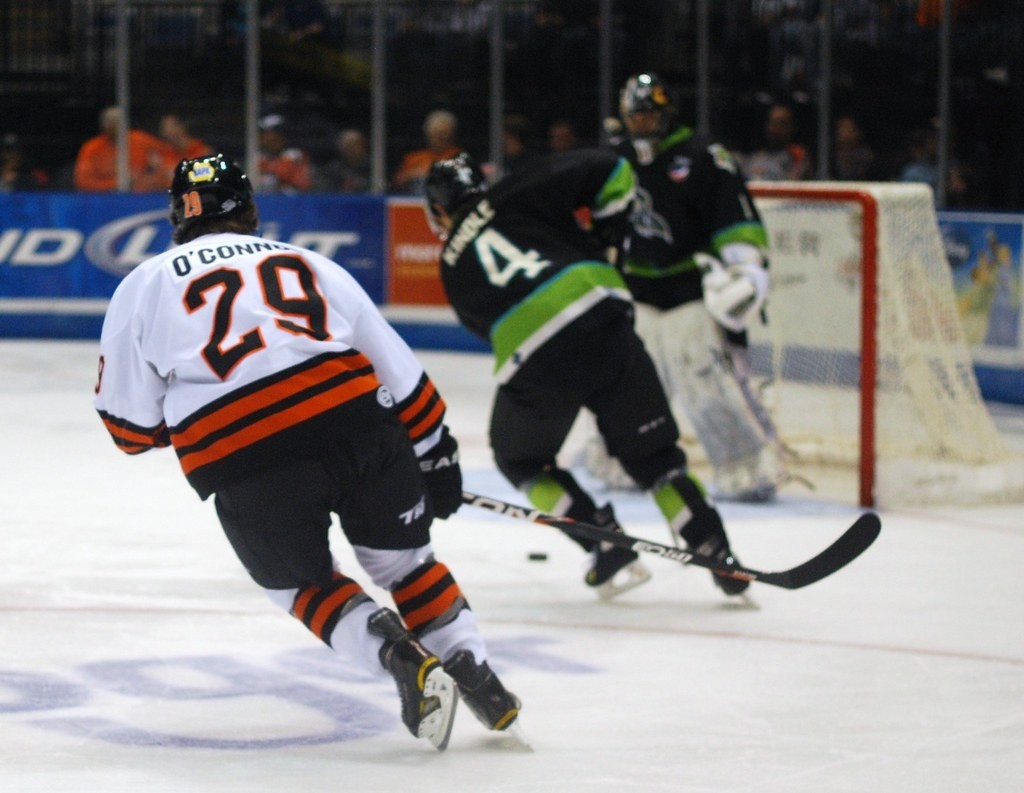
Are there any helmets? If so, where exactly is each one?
[168,153,255,232]
[618,72,681,142]
[427,153,490,234]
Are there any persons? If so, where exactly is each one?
[587,73,818,504]
[93,152,522,751]
[0,0,1024,216]
[421,147,754,609]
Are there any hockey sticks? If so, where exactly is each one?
[461,491,881,591]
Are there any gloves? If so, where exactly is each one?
[418,426,462,519]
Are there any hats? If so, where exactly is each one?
[258,115,291,133]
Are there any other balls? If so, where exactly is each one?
[527,550,548,561]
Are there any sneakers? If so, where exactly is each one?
[695,535,760,609]
[445,648,535,752]
[366,607,456,749]
[587,502,652,601]
